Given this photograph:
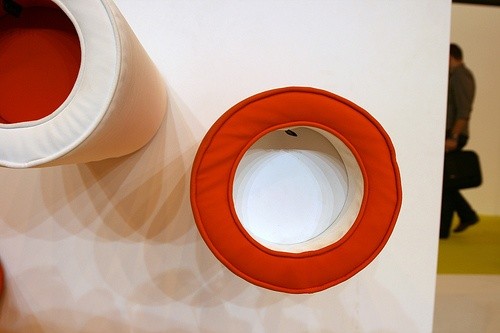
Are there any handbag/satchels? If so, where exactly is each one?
[444,144,482,189]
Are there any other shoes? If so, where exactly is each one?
[440,229,451,239]
[453,213,479,233]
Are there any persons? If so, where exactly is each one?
[439,42,481,240]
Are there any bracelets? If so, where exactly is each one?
[450,134,458,139]
